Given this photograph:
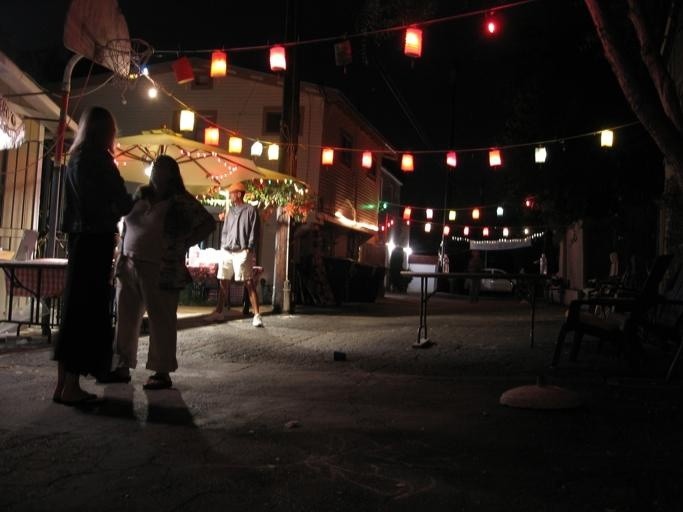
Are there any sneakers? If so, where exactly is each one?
[53,380,98,405]
[203,311,225,322]
[252,314,263,328]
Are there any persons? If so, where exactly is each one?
[95,154,216,391]
[52,104,134,405]
[201,181,263,328]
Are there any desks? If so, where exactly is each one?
[0,256,70,346]
[184,259,264,307]
[398,268,546,351]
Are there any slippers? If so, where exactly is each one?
[144,372,177,390]
[96,367,134,385]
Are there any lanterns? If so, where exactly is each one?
[402,27,424,59]
[204,126,220,146]
[171,54,196,86]
[402,196,534,238]
[209,51,228,80]
[268,46,287,74]
[179,109,195,132]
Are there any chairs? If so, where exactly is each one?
[545,244,683,387]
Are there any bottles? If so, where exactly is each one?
[442,254,449,273]
[539,253,548,276]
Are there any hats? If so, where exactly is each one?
[228,182,245,193]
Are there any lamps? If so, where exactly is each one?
[181,107,280,162]
[268,42,288,71]
[141,152,312,222]
[143,67,160,100]
[324,146,415,175]
[403,24,424,57]
[168,40,230,85]
[443,146,548,169]
[335,38,355,74]
[377,198,531,239]
[601,129,614,149]
[482,10,500,37]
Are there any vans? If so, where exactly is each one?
[480,268,514,294]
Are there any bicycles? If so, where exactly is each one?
[543,275,616,368]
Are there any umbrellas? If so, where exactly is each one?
[50,121,308,196]
